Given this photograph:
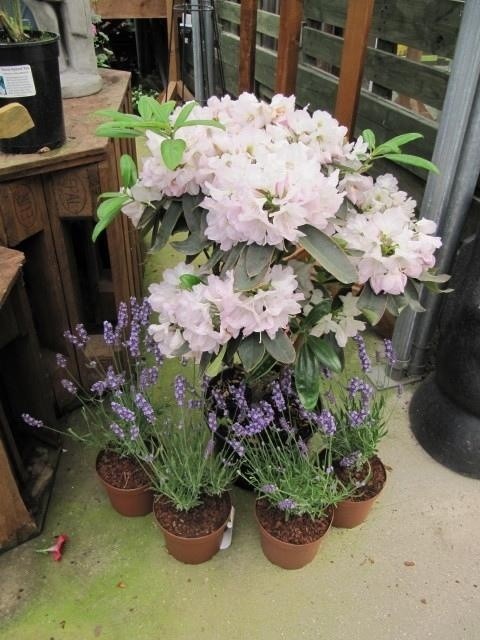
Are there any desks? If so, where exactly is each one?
[1,247,62,554]
[1,67,147,418]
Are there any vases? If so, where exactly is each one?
[95,433,166,517]
[203,362,323,491]
[254,486,335,569]
[331,452,388,529]
[0,30,67,155]
[152,480,231,564]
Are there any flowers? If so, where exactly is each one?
[89,84,455,412]
